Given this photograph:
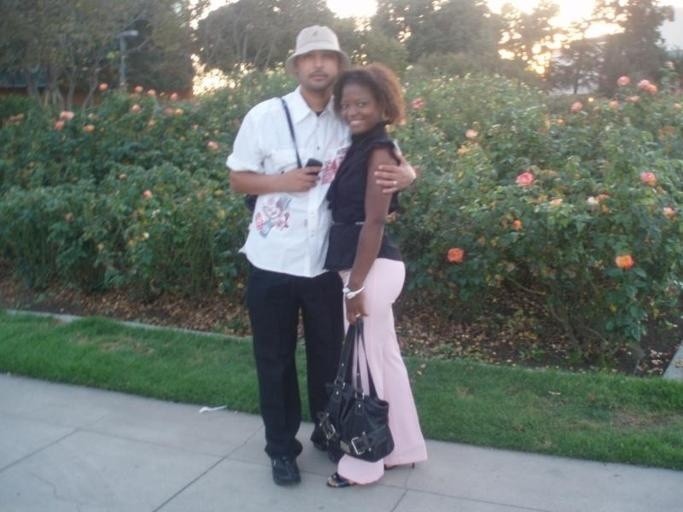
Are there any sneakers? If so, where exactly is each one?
[270,453,300,485]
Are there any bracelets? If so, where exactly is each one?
[343,280,365,299]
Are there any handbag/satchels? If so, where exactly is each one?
[310,318,394,462]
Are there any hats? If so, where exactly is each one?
[284,26,347,75]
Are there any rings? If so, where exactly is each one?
[392,180,398,188]
[355,313,361,318]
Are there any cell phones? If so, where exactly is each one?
[305,158,322,175]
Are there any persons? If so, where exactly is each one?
[226,23,418,485]
[323,61,428,488]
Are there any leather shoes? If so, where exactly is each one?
[327,472,355,487]
